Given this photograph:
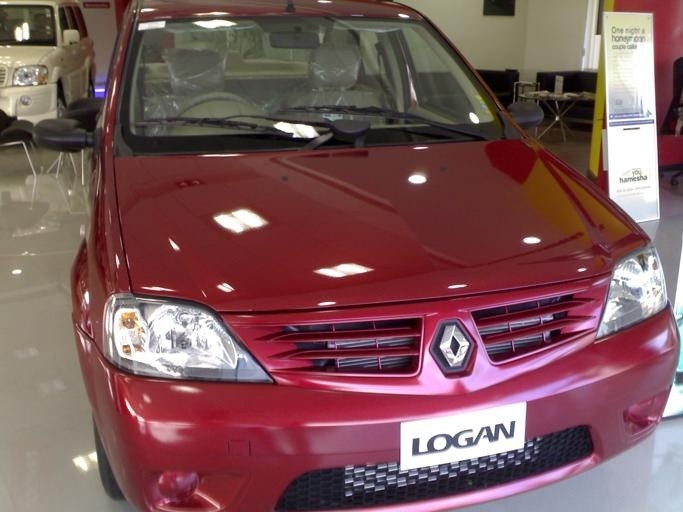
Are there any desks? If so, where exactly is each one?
[518,90,589,144]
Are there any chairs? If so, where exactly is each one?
[0,98,103,187]
[282,42,384,125]
[507,81,543,139]
[144,49,234,136]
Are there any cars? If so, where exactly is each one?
[64,1,683,512]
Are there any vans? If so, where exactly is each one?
[1,0,100,140]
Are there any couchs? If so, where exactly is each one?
[413,68,520,124]
[536,69,596,120]
